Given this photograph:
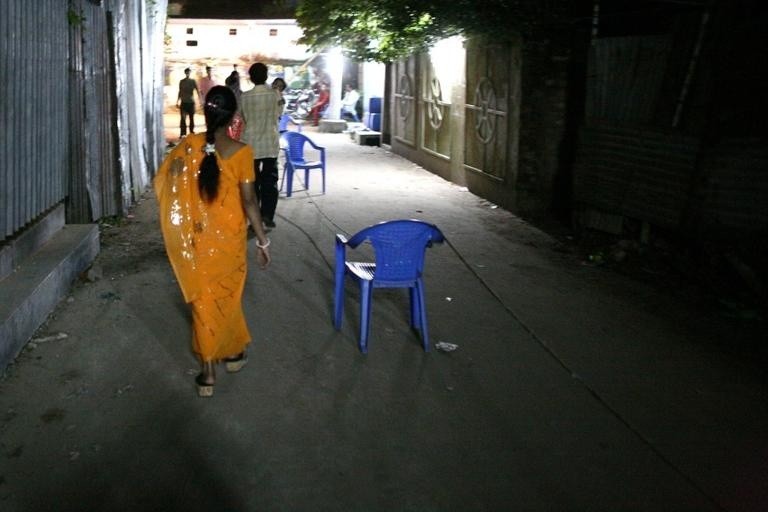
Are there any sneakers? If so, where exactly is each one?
[261,217,276,228]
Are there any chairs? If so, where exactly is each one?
[279,115,303,133]
[283,93,311,120]
[278,132,325,197]
[332,220,445,354]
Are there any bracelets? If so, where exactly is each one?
[255,237,271,248]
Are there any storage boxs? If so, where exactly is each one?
[362,97,381,131]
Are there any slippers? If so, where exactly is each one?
[225,354,248,373]
[196,373,214,398]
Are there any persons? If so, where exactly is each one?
[309,83,360,126]
[177,63,287,228]
[152,85,272,397]
